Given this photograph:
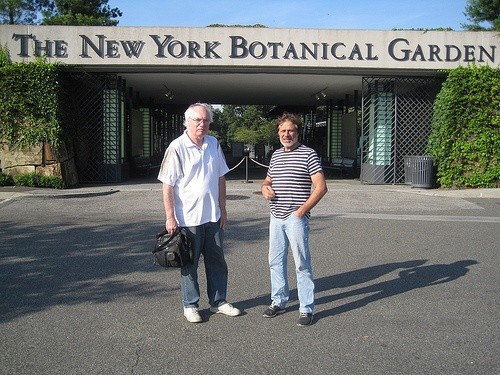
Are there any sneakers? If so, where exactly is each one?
[209,302,242,316]
[183,306,203,322]
[296,312,314,327]
[262,301,286,318]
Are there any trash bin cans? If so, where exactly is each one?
[402,155,434,188]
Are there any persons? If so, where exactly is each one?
[260,113,328,328]
[157,103,243,323]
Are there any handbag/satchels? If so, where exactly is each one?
[152,226,195,268]
